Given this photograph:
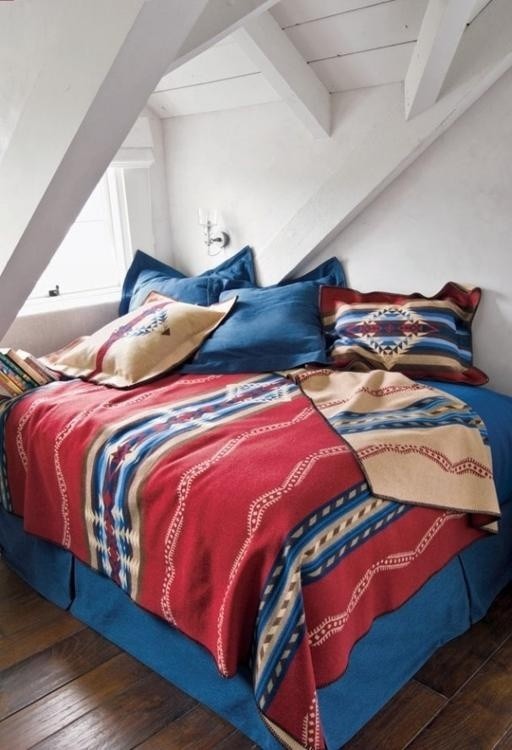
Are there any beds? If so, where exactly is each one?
[0,354,511,750]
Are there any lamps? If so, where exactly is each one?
[197,207,232,257]
